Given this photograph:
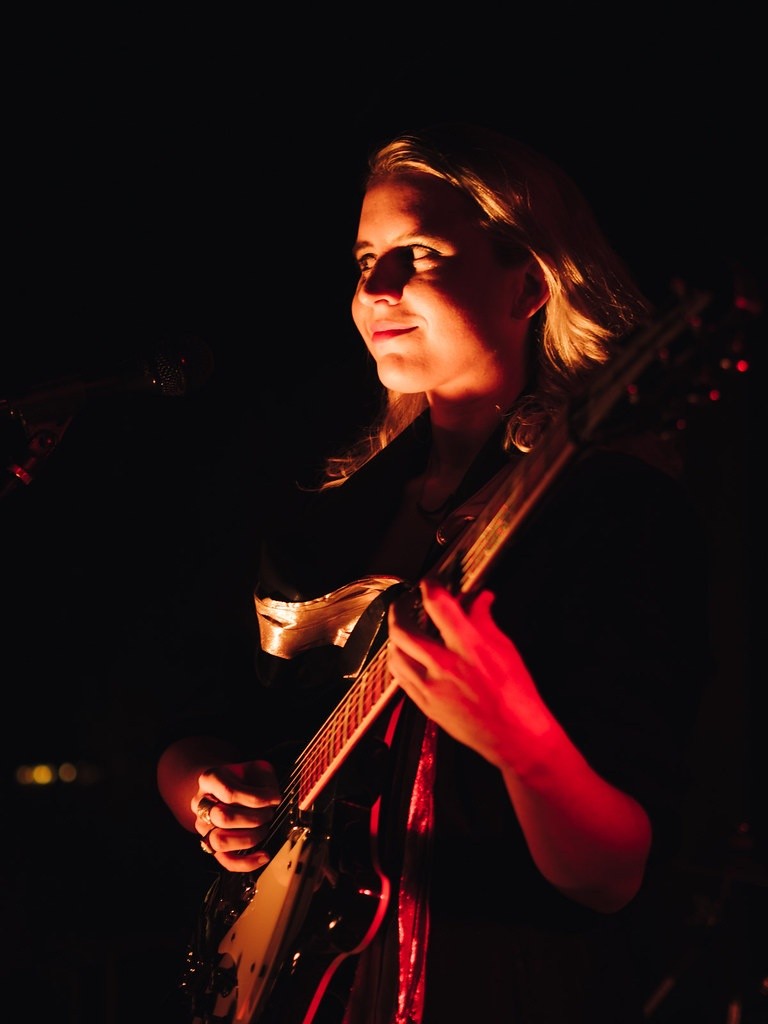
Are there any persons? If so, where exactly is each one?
[157,126,760,1024]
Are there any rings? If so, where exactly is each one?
[200,827,216,855]
[197,794,219,825]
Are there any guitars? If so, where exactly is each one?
[182,286,721,1024]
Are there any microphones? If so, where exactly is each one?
[0,333,215,415]
